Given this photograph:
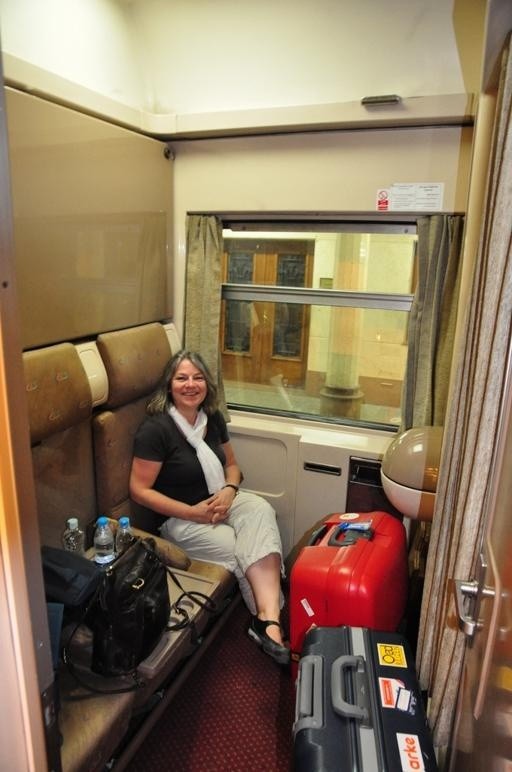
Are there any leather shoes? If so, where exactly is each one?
[248,614,290,663]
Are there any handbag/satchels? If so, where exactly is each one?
[94,535,171,676]
[40,542,103,625]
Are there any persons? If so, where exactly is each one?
[128,348,292,665]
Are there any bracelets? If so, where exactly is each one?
[221,483,239,491]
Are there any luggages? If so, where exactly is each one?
[293,625,438,771]
[289,509,408,701]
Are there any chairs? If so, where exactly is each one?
[21,323,198,772]
[91,322,229,633]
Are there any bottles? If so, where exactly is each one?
[62,517,133,565]
